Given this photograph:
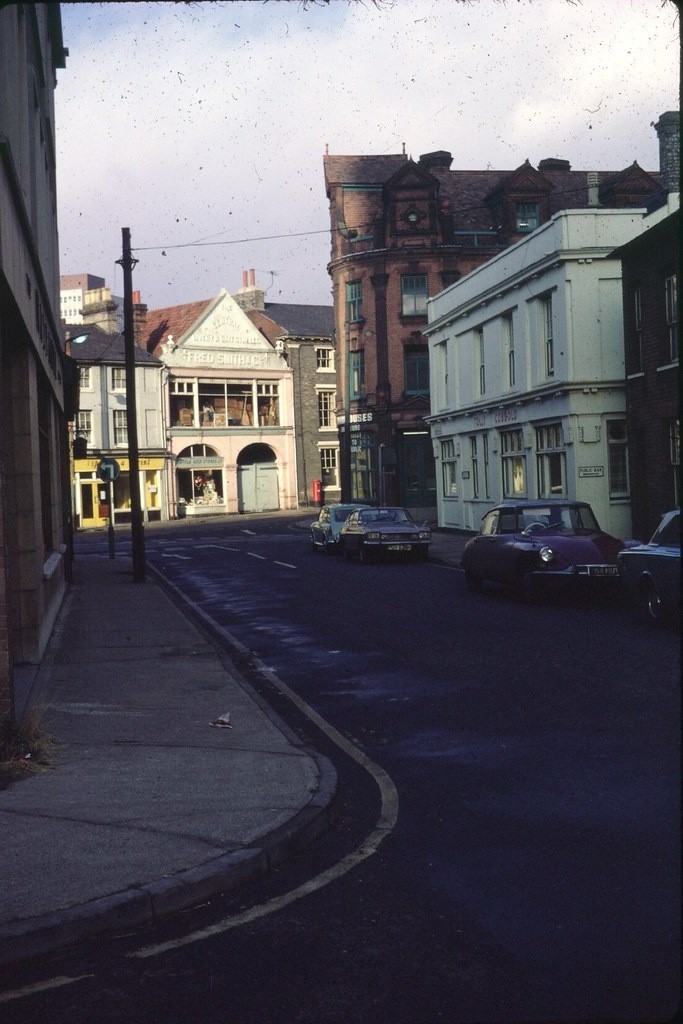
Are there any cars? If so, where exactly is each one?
[461,498,625,603]
[310,504,372,555]
[616,510,683,626]
[339,506,433,567]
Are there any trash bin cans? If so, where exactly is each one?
[313,479,321,507]
